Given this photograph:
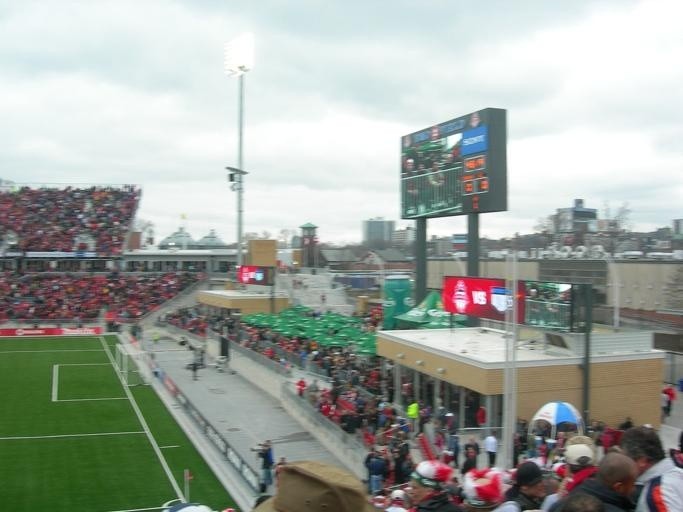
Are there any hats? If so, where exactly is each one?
[391,443,594,503]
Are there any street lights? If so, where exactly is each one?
[224,62,251,267]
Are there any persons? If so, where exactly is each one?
[0,255,208,326]
[157,304,383,377]
[0,180,140,255]
[523,280,574,330]
[402,141,463,218]
